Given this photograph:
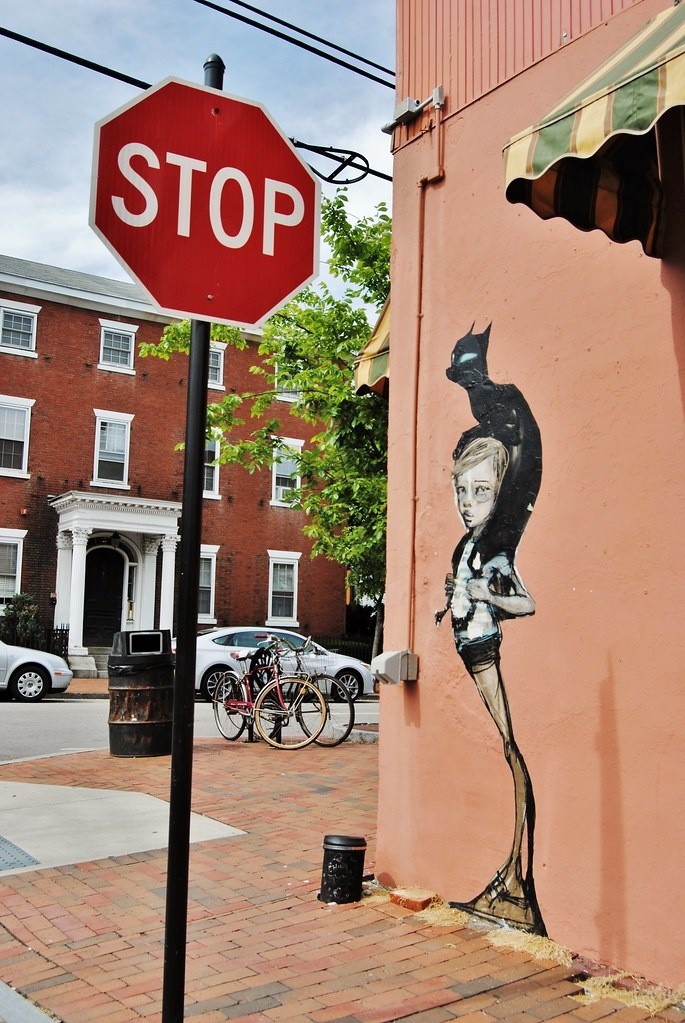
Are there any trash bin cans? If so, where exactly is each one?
[106,630,174,757]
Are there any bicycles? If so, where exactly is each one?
[210,631,356,750]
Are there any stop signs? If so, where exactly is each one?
[88,81,325,338]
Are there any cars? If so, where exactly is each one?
[169,626,374,701]
[1,638,74,702]
